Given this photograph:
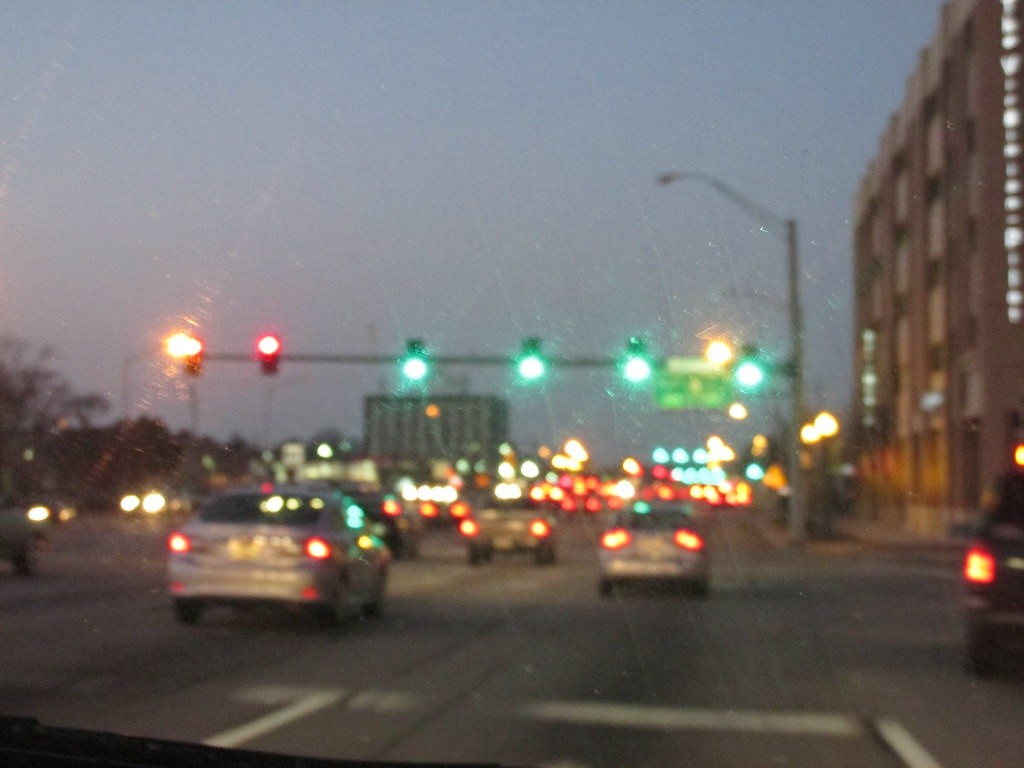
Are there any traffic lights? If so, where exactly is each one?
[515,339,543,382]
[255,333,281,376]
[170,333,205,375]
[734,344,762,389]
[624,337,649,385]
[403,338,428,385]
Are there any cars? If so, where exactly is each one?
[1,498,54,577]
[161,485,394,631]
[466,495,558,569]
[596,501,711,599]
[344,488,422,562]
[952,469,1024,666]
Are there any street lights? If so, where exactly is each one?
[658,166,808,546]
